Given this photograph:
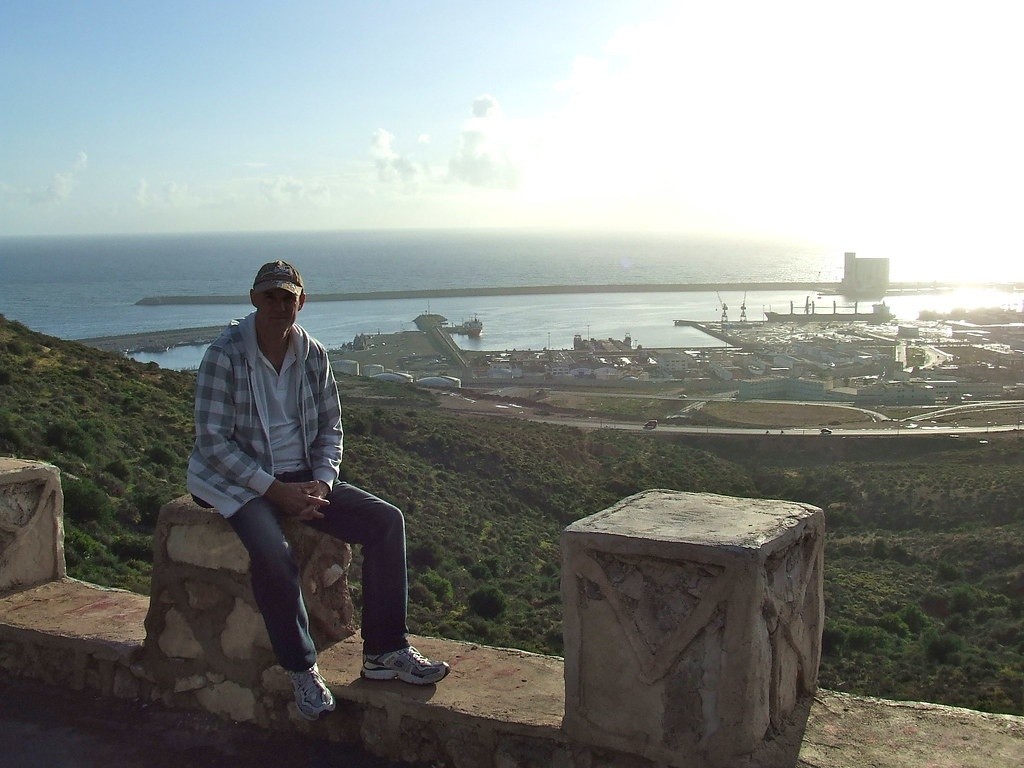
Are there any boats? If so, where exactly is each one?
[762,297,896,323]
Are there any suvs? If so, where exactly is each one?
[821,429,832,434]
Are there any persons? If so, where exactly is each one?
[184,262,451,723]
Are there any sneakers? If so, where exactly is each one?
[290,662,335,722]
[360,645,453,686]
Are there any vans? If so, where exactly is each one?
[644,420,657,426]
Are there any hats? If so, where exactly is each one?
[253,260,304,297]
